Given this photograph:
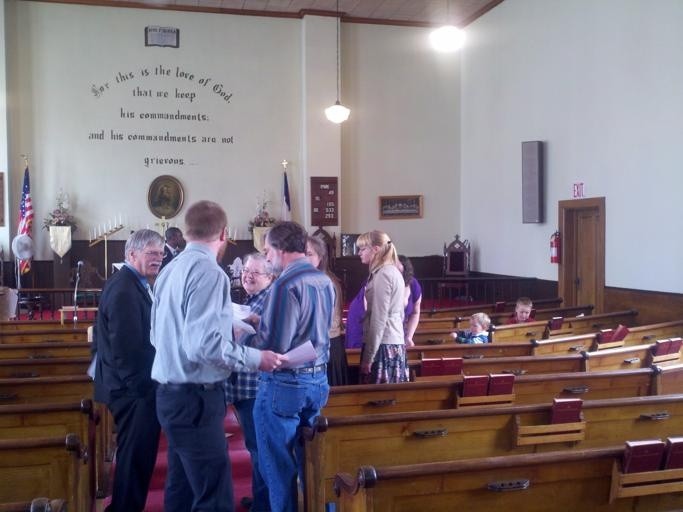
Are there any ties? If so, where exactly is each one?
[146,282,153,303]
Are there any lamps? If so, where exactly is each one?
[325,0,350,123]
[426,0,467,55]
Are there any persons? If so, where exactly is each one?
[450,312,491,358]
[506,296,537,325]
[305,236,350,386]
[232,221,336,511]
[152,201,291,512]
[356,230,411,385]
[344,255,422,348]
[90,230,166,511]
[160,227,183,269]
[229,252,273,512]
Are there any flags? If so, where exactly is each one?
[282,172,291,221]
[16,166,35,275]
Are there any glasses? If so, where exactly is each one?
[146,250,167,259]
[241,268,267,276]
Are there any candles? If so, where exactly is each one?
[89,213,122,243]
[162,216,165,224]
[227,226,237,242]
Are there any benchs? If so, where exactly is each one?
[302,298,683,512]
[0,307,118,512]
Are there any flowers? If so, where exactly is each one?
[41,207,79,232]
[248,211,276,232]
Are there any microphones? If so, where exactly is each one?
[76,260,84,278]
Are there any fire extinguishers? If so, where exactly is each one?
[550,232,561,263]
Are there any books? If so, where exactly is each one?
[421,357,515,406]
[654,337,682,362]
[664,436,683,470]
[623,439,665,473]
[550,398,583,423]
[599,324,630,344]
[530,309,536,319]
[495,302,505,314]
[551,317,563,330]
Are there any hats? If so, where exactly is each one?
[11,233,35,259]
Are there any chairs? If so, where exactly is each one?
[312,226,348,303]
[437,234,470,304]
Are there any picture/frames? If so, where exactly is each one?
[378,194,424,220]
[148,175,184,219]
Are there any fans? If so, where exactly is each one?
[11,233,36,297]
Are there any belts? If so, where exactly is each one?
[279,363,326,374]
